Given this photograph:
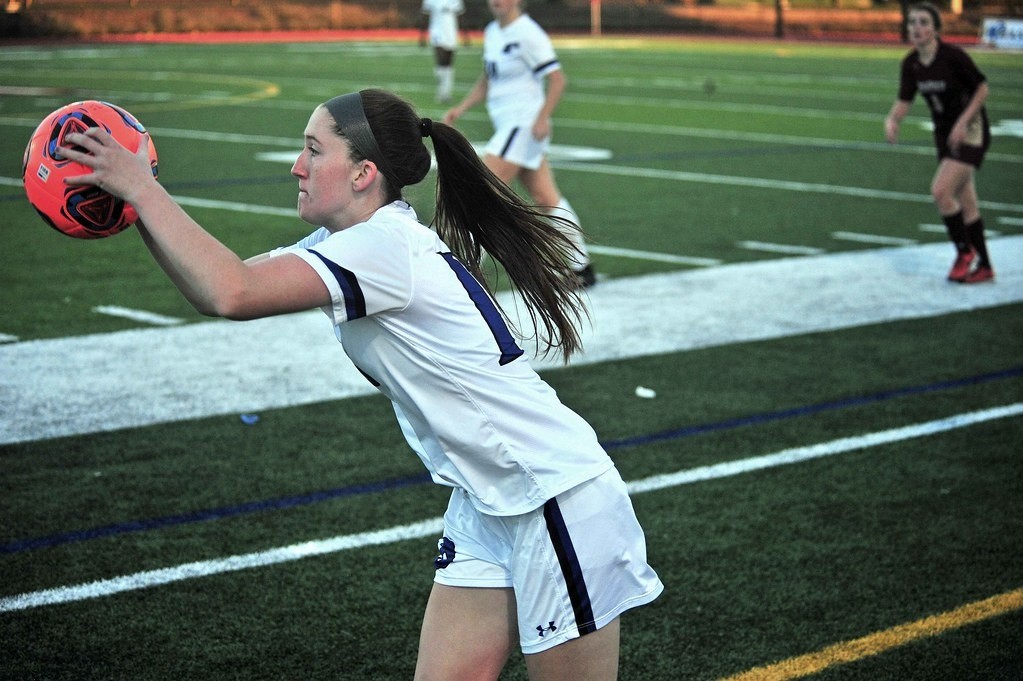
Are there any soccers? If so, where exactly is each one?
[21,99,160,241]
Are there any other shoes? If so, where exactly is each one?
[560,263,594,290]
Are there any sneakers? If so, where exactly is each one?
[949,242,980,279]
[962,267,994,282]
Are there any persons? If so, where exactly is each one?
[884,3,994,284]
[443,0,596,290]
[55,88,665,681]
[421,0,467,104]
